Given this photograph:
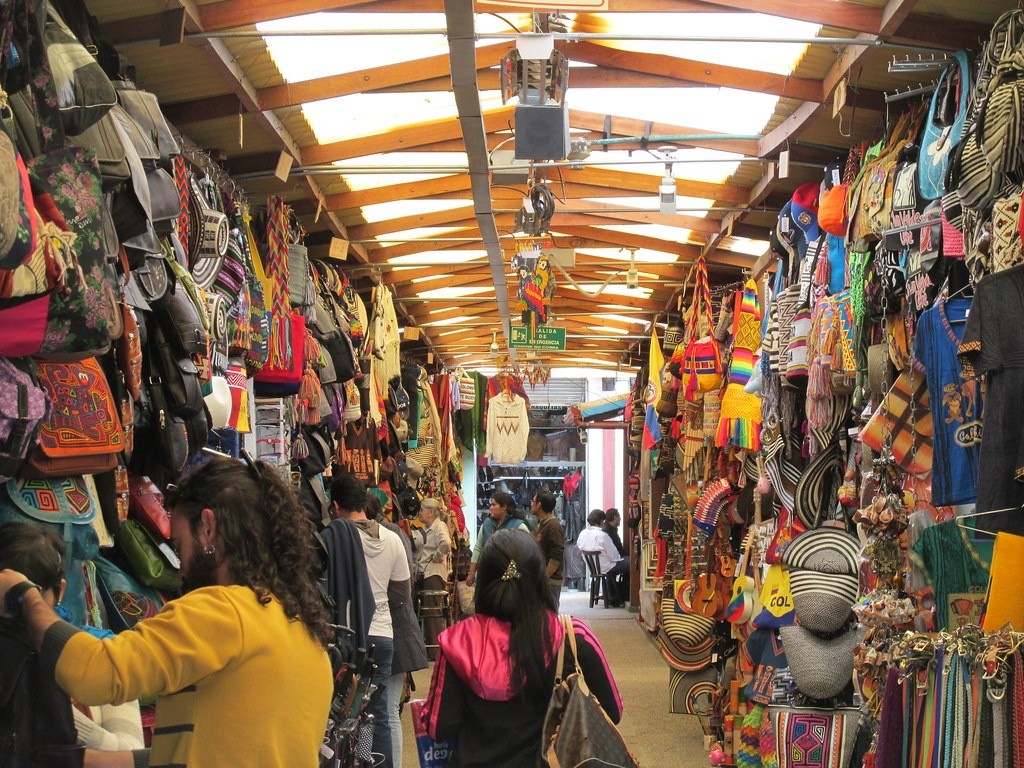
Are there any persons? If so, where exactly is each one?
[0,456,336,768]
[320,475,428,768]
[531,492,565,611]
[419,528,624,767]
[418,499,452,663]
[465,492,531,587]
[0,521,146,768]
[576,508,631,605]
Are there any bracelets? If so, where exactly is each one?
[465,571,476,576]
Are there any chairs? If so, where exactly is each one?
[580,550,621,609]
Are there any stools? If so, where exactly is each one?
[416,590,452,648]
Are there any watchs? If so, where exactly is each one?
[4,580,41,622]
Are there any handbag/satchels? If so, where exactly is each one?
[542,615,639,768]
[625,0,1024,768]
[410,699,454,768]
[0,0,474,632]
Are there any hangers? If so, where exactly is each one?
[175,135,253,206]
[955,504,1024,543]
[501,389,513,403]
[377,267,385,286]
[721,281,745,297]
[946,278,974,324]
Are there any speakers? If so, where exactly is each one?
[512,103,570,161]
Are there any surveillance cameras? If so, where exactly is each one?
[580,431,588,444]
